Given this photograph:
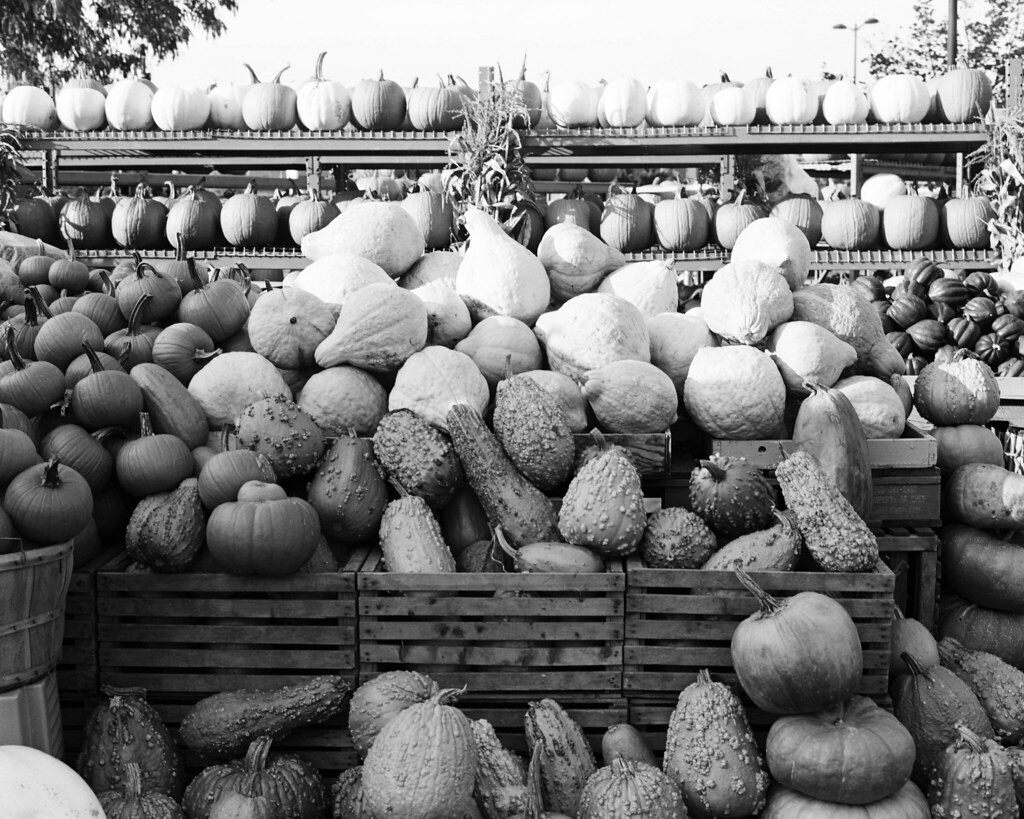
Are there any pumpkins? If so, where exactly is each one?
[0,44,1024,819]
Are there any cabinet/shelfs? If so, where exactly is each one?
[0,66,991,270]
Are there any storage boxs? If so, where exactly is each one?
[572,421,941,637]
[63,537,892,736]
[0,537,76,690]
[1,670,66,762]
